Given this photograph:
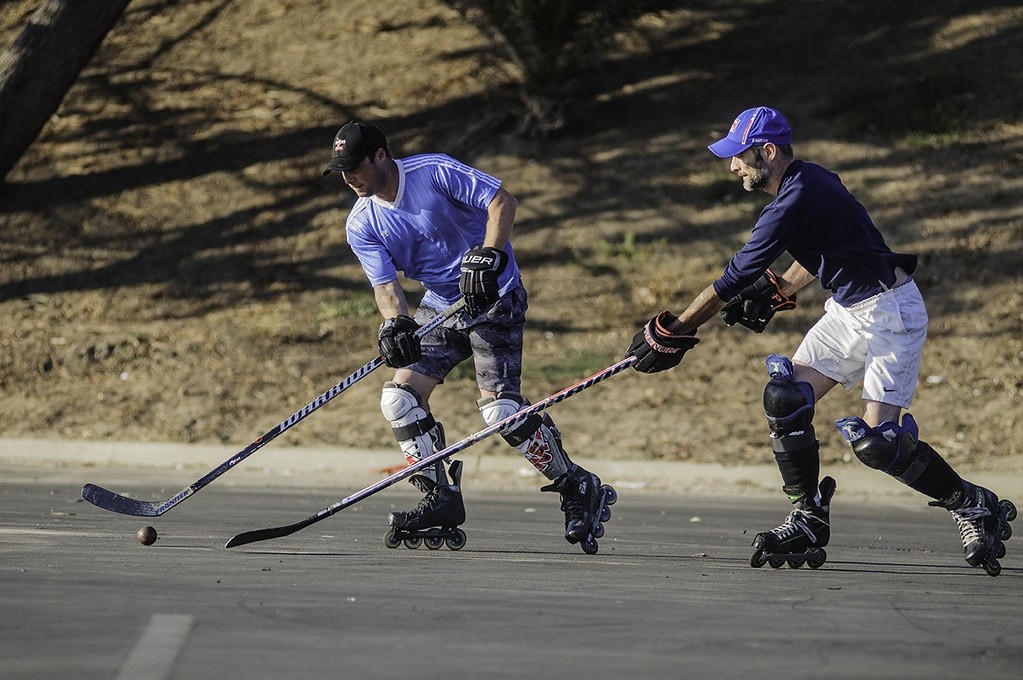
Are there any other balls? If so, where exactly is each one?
[137,526,157,545]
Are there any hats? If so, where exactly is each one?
[321,119,387,177]
[706,105,793,160]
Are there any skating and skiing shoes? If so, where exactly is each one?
[748,475,837,570]
[927,477,1017,577]
[539,448,618,556]
[381,459,467,551]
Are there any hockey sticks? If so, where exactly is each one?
[81,293,468,518]
[224,352,639,549]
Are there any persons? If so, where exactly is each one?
[320,119,616,556]
[625,105,1018,578]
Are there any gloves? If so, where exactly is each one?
[457,246,509,320]
[624,309,701,374]
[720,267,799,334]
[377,314,422,369]
